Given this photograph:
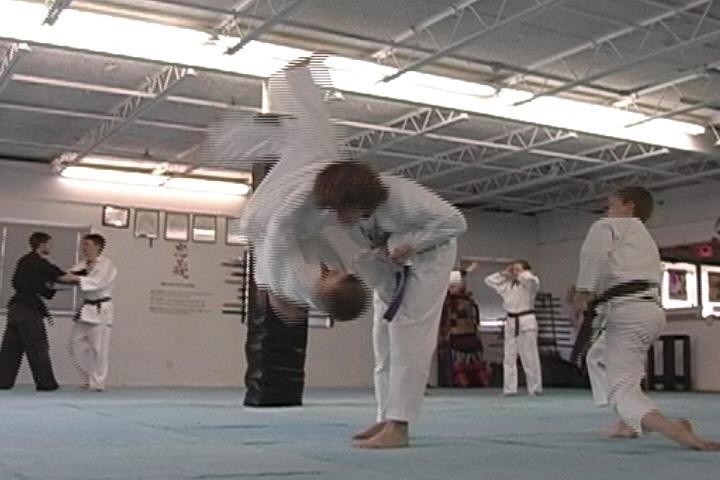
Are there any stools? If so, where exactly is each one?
[647,335,692,391]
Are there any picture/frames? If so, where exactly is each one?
[102,205,249,245]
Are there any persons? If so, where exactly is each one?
[314,159,466,448]
[56,234,118,392]
[0,232,96,391]
[484,260,543,397]
[565,186,720,451]
[214,52,386,322]
